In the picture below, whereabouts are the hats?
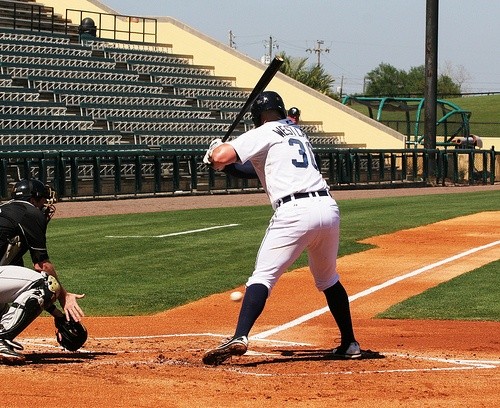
[288,107,300,117]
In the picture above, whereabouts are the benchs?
[0,0,377,180]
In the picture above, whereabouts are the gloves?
[203,138,225,171]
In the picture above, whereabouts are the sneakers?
[332,338,362,358]
[203,335,248,365]
[0,338,25,365]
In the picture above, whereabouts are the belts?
[278,191,332,207]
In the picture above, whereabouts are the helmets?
[11,177,52,199]
[251,91,287,128]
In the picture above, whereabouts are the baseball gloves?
[54,312,88,351]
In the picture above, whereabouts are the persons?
[288,107,300,124]
[0,178,85,364]
[199,91,362,367]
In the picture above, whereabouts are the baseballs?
[230,291,243,302]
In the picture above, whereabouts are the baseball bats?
[207,56,285,169]
